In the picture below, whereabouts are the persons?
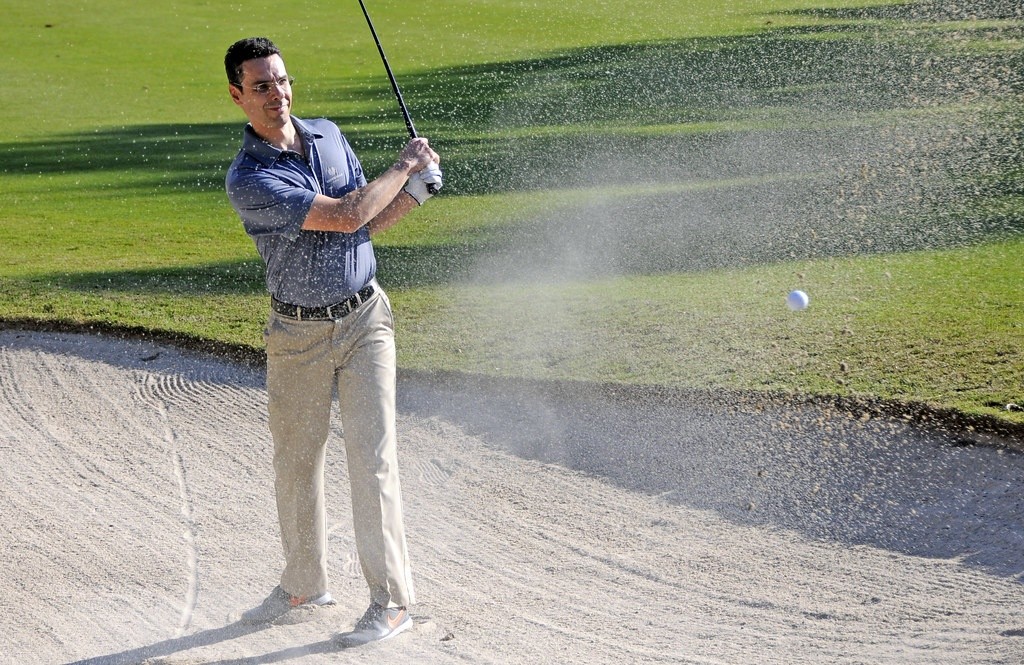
[225,38,443,648]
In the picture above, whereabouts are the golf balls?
[787,290,809,311]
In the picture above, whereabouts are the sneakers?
[340,600,411,647]
[242,581,332,626]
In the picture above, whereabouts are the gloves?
[404,159,443,206]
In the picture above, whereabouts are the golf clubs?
[358,0,442,196]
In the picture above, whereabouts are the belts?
[271,286,375,320]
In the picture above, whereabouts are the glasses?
[230,75,298,99]
